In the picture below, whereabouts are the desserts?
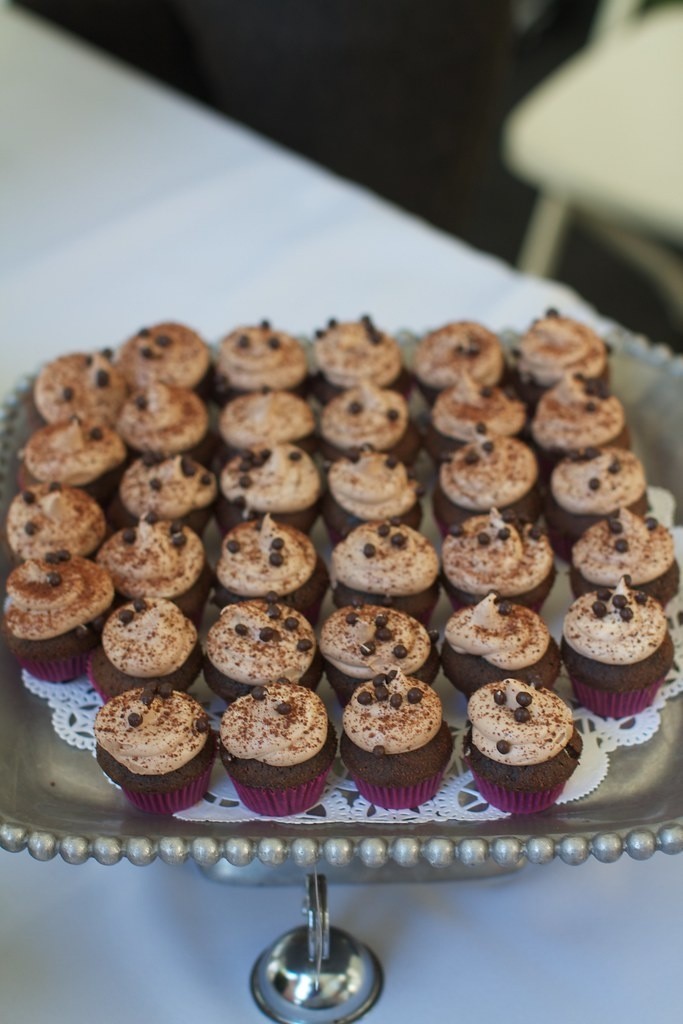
[0,307,680,813]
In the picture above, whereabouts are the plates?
[0,374,683,867]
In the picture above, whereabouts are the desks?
[0,0,683,1024]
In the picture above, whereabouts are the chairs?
[499,0,683,310]
